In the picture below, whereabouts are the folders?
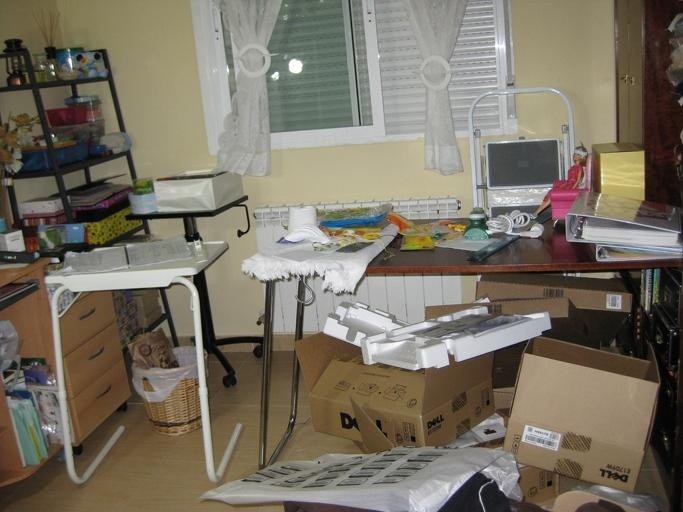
[565,190,682,247]
[595,244,683,263]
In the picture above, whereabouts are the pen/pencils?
[123,246,130,266]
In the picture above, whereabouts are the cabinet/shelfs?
[613,0,683,207]
[59,290,132,444]
[0,48,179,352]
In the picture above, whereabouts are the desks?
[43,241,243,486]
[124,193,248,389]
[248,203,682,471]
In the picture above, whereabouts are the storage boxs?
[503,336,661,494]
[154,168,243,212]
[76,200,143,244]
[472,406,560,503]
[424,298,598,386]
[592,142,645,200]
[295,332,496,447]
[476,272,633,313]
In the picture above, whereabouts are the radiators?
[252,196,462,336]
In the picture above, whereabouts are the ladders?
[467,85,577,284]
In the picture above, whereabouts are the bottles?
[33,52,47,83]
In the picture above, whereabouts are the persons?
[529,145,588,220]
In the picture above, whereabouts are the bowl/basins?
[45,107,87,128]
